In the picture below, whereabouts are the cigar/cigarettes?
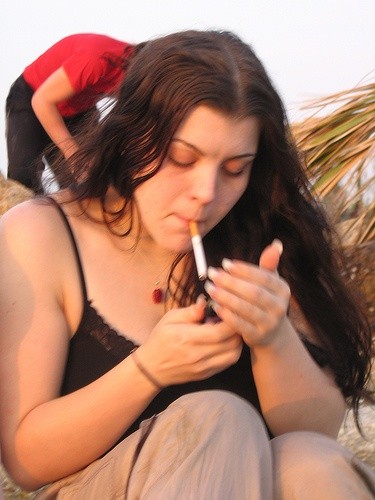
[189,221,208,280]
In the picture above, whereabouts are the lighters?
[205,298,220,323]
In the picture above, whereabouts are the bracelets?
[129,347,165,391]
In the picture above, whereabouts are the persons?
[5,33,136,195]
[0,29,375,500]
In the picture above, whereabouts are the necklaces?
[113,201,176,304]
